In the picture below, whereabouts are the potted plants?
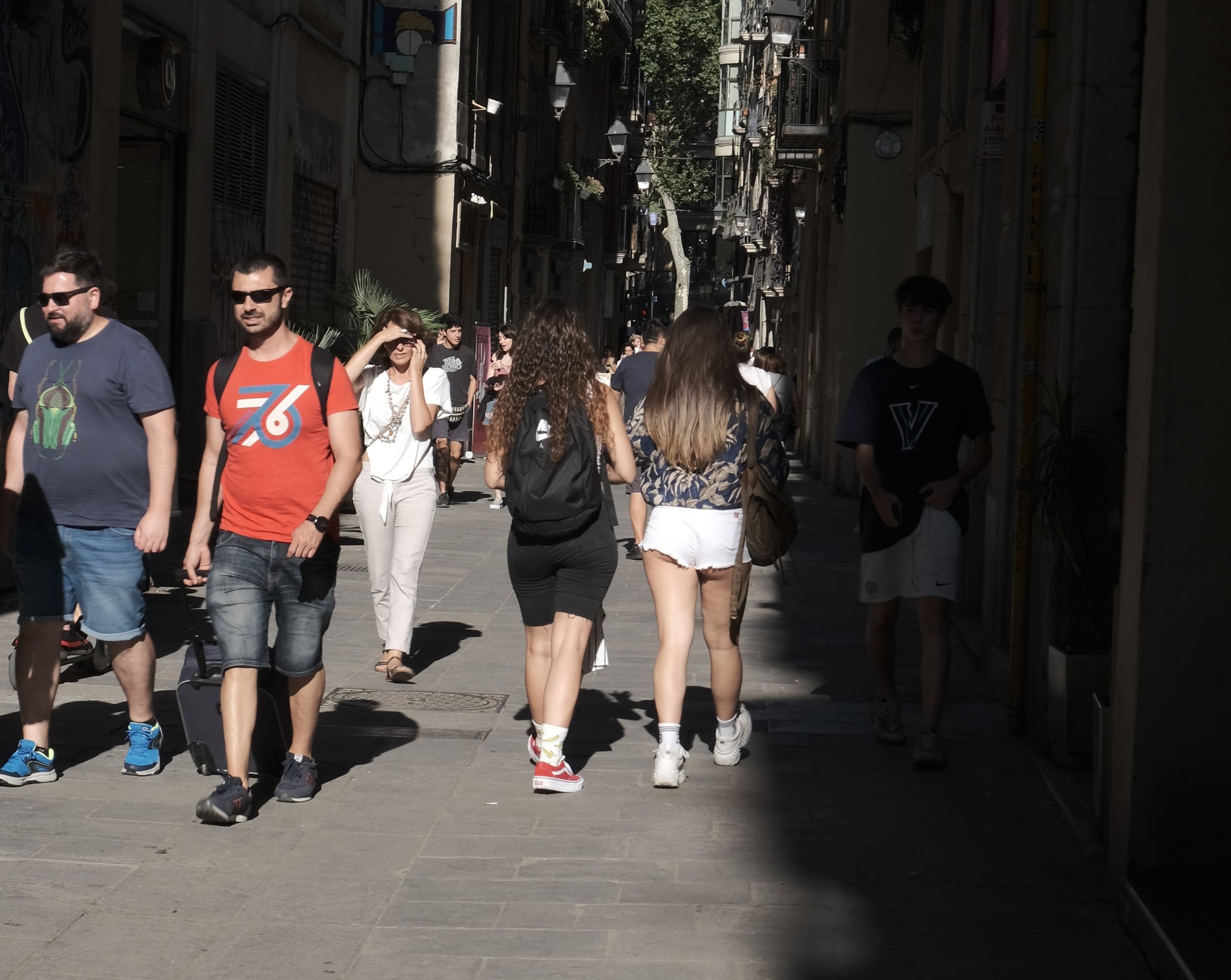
[647,201,664,226]
[553,163,580,190]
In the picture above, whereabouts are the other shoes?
[625,543,643,560]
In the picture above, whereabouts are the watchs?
[307,514,330,533]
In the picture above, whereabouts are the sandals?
[386,655,414,681]
[374,644,388,673]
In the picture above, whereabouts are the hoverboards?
[8,613,111,693]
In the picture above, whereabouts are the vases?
[580,188,591,201]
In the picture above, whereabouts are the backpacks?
[503,374,620,539]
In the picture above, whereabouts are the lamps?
[514,58,578,132]
[712,204,725,227]
[633,157,655,197]
[581,114,631,172]
[709,269,718,285]
[471,98,504,115]
[732,206,758,235]
[762,1,840,81]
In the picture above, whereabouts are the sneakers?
[489,497,503,509]
[196,768,253,823]
[446,486,457,504]
[652,741,690,788]
[436,489,450,508]
[0,739,58,787]
[527,732,583,793]
[121,719,164,777]
[274,751,318,803]
[60,632,94,655]
[911,722,947,770]
[714,701,752,767]
[869,695,907,745]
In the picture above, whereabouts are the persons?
[182,251,363,823]
[732,332,802,648]
[343,305,452,682]
[625,297,789,794]
[0,248,176,788]
[833,277,995,772]
[484,297,636,791]
[594,315,668,560]
[423,312,520,510]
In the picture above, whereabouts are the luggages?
[174,570,296,778]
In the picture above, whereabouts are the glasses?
[229,284,290,305]
[384,336,416,348]
[36,285,97,307]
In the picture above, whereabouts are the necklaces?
[380,370,415,442]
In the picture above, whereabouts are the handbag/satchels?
[445,403,468,423]
[729,384,800,620]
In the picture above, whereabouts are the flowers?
[583,176,604,200]
